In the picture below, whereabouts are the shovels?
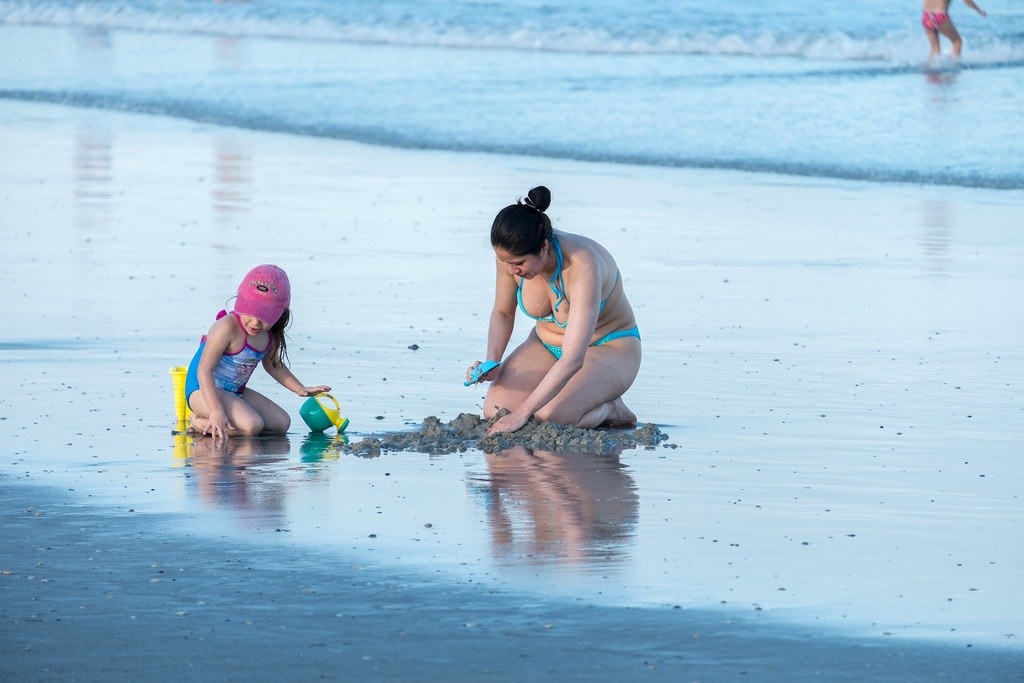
[464,360,501,387]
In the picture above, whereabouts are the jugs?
[300,392,351,433]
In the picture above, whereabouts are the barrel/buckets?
[170,364,192,421]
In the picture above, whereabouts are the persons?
[184,264,332,439]
[922,0,987,57]
[465,186,643,437]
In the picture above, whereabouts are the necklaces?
[531,251,557,324]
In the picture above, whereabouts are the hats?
[234,264,291,325]
